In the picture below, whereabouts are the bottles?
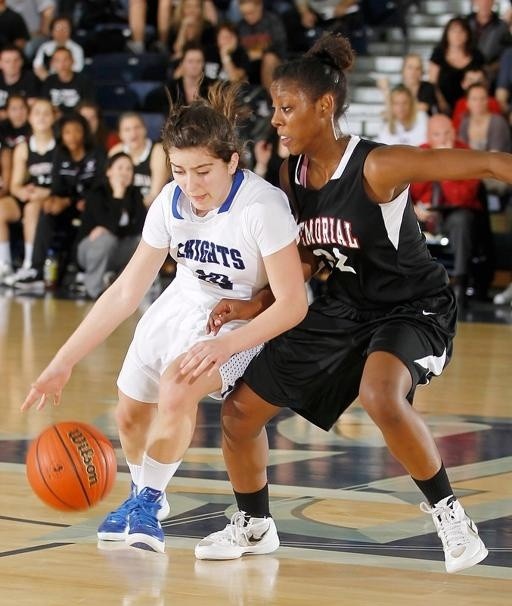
[43,249,58,287]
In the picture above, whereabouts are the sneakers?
[0,263,50,294]
[195,509,279,561]
[98,482,169,555]
[419,495,489,573]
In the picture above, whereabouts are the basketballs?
[26,423,117,511]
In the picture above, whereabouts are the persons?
[194,30,511,574]
[2,0,510,304]
[19,70,309,556]
